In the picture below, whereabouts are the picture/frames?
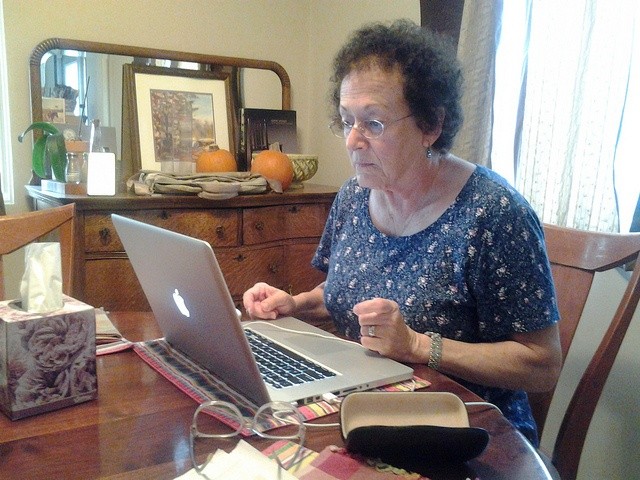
[121,62,237,176]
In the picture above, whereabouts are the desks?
[0,295,551,480]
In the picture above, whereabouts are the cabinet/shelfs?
[20,179,343,311]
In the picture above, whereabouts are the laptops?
[111,213,414,411]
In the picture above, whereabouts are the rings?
[369,325,375,338]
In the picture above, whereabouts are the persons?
[243,19,563,449]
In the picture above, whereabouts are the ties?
[328,112,415,140]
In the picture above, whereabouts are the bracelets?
[424,332,442,372]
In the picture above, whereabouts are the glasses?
[190,400,306,472]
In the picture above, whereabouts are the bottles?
[82,152,88,183]
[89,119,105,152]
[65,152,82,184]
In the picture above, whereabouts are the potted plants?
[17,120,91,182]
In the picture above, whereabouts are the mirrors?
[29,35,293,182]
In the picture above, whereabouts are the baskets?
[251,151,318,189]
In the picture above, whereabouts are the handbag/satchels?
[126,169,283,200]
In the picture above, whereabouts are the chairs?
[1,203,79,303]
[521,218,639,477]
